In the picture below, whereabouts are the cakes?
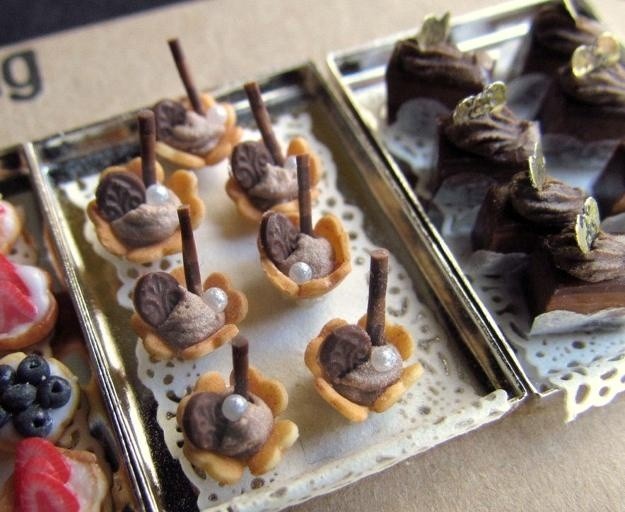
[128,204,249,361]
[0,349,80,453]
[594,143,625,219]
[424,80,543,195]
[0,251,58,353]
[385,10,497,128]
[256,153,352,301]
[225,81,321,221]
[86,110,205,263]
[518,195,625,319]
[0,436,112,511]
[152,37,243,168]
[468,143,600,256]
[177,335,300,485]
[521,1,625,75]
[537,29,624,123]
[0,196,40,267]
[304,249,425,424]
